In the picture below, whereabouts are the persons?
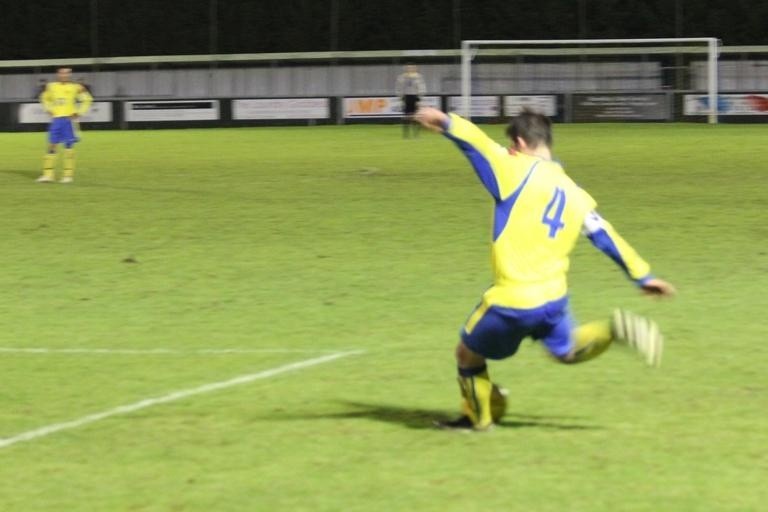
[395,66,427,139]
[414,105,678,429]
[35,65,94,183]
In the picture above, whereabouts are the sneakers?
[433,416,496,430]
